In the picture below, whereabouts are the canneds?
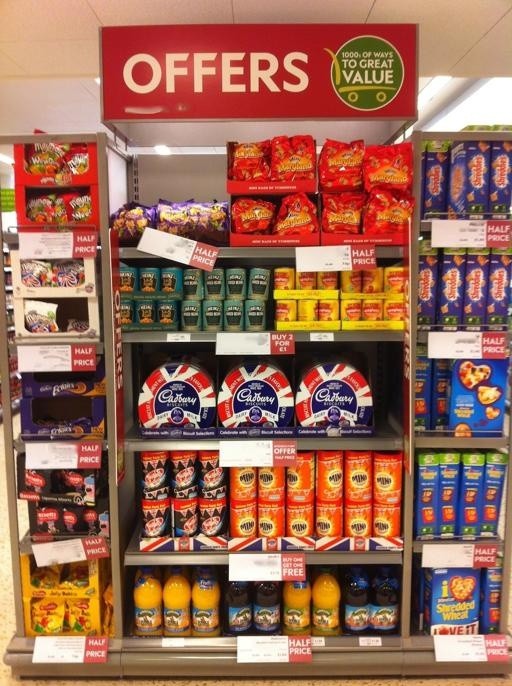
[119,265,406,332]
[141,451,401,539]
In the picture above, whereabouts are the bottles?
[132,568,399,636]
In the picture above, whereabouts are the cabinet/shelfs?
[0,128,511,681]
